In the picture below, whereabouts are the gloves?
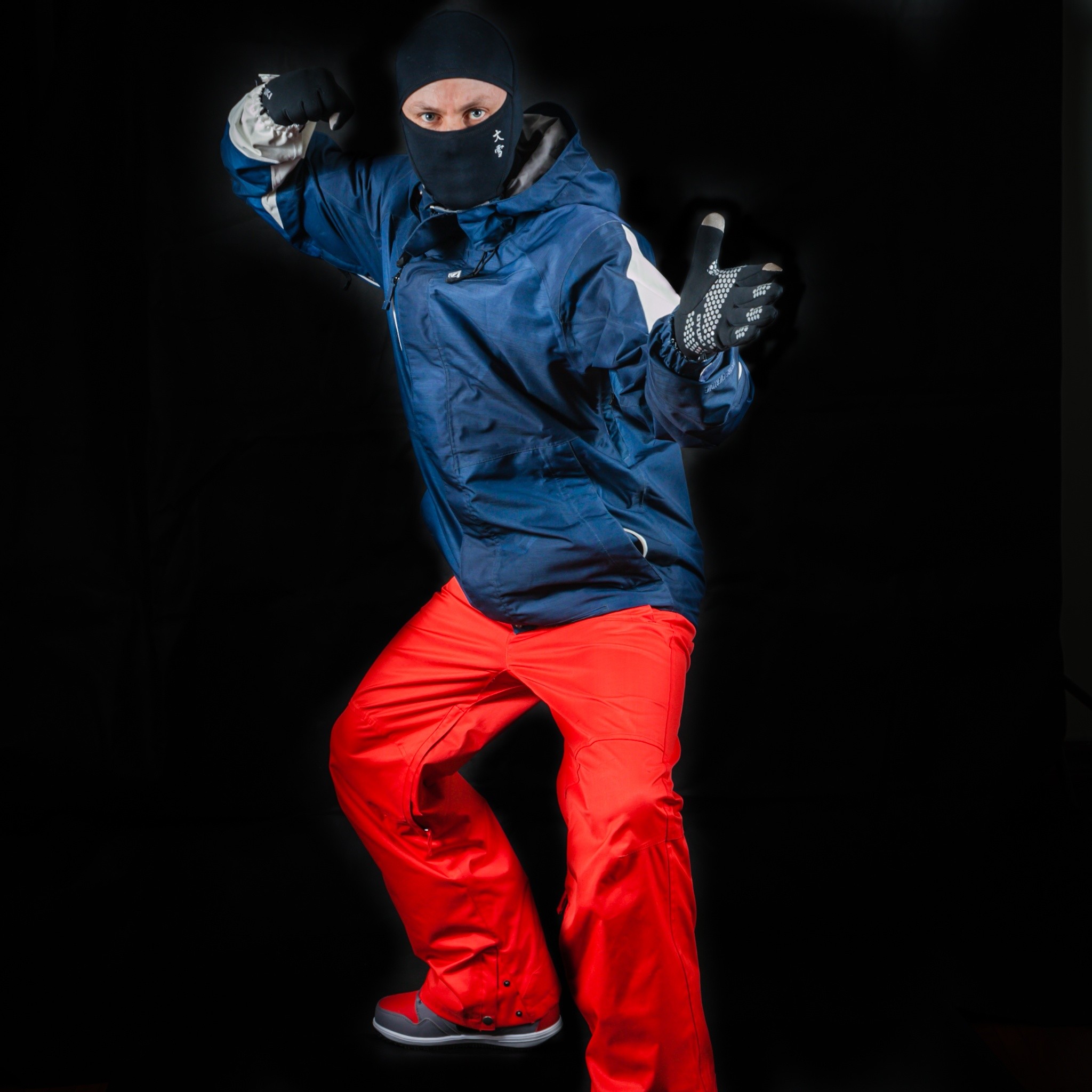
[673,211,785,360]
[262,67,351,131]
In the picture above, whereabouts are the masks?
[399,92,522,210]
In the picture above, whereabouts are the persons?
[219,9,783,1092]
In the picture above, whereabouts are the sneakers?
[372,989,564,1047]
